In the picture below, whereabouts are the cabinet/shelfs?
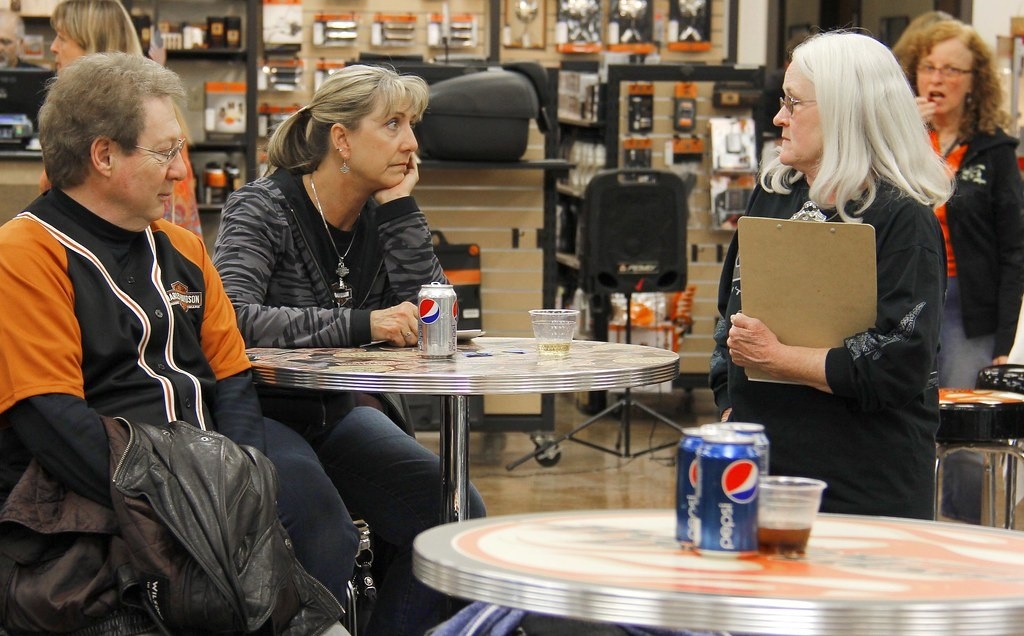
[121,0,258,211]
[555,64,762,414]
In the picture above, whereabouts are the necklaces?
[310,172,362,275]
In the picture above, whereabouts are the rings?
[403,331,412,337]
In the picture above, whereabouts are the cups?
[757,476,828,557]
[529,309,580,359]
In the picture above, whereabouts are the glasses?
[89,138,187,166]
[915,60,977,79]
[779,95,817,115]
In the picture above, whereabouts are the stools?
[932,388,1024,530]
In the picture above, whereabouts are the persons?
[0,11,42,73]
[213,65,488,636]
[1,53,353,636]
[711,31,954,521]
[38,0,203,238]
[892,9,1024,520]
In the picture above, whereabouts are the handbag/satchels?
[423,600,733,636]
[430,229,482,337]
[340,517,379,635]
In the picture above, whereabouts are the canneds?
[417,281,458,357]
[674,421,770,558]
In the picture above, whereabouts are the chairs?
[506,167,697,471]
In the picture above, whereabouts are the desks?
[411,508,1024,636]
[245,336,681,528]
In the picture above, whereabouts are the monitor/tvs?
[0,67,55,131]
